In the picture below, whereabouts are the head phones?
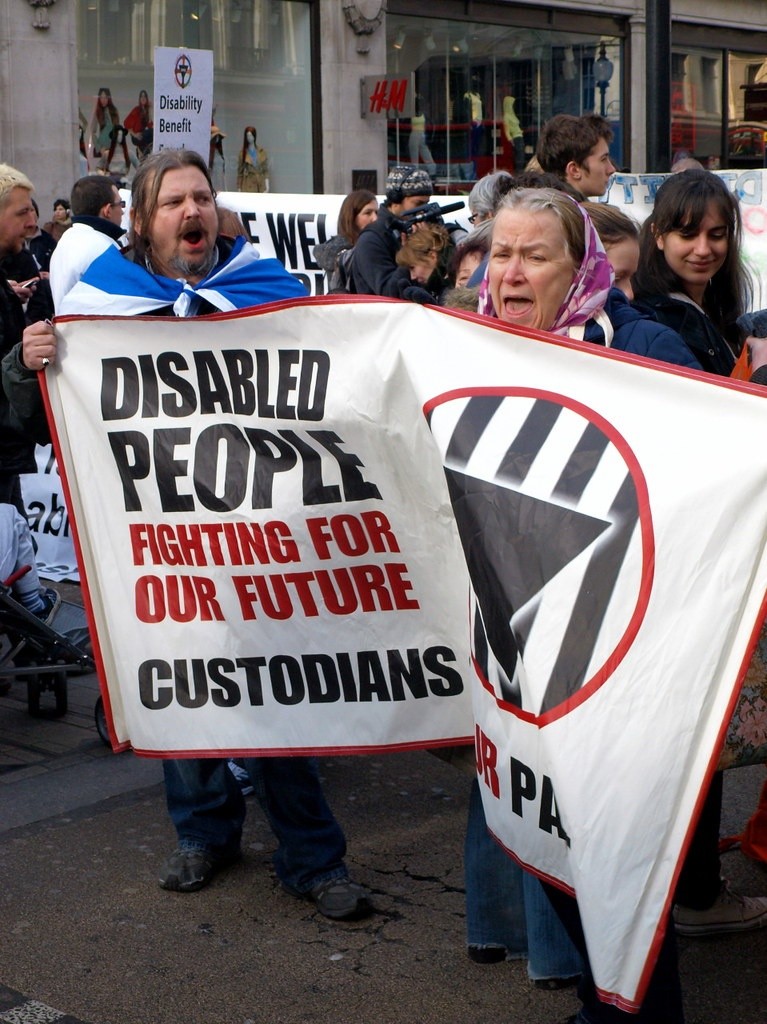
[387,166,416,205]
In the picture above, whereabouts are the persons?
[407,75,536,179]
[78,81,270,191]
[0,115,767,1023]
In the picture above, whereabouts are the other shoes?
[39,586,62,627]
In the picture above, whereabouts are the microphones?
[412,201,465,221]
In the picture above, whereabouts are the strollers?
[0,565,114,751]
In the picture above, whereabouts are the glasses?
[100,200,126,208]
[468,213,478,224]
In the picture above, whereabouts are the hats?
[386,166,432,197]
[31,198,39,217]
[210,126,226,139]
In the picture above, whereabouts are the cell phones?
[22,280,38,289]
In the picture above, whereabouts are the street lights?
[593,40,615,118]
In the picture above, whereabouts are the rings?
[42,358,49,367]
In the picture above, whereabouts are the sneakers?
[672,876,767,935]
[158,847,214,893]
[228,761,253,795]
[284,876,370,920]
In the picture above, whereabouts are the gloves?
[389,272,438,305]
